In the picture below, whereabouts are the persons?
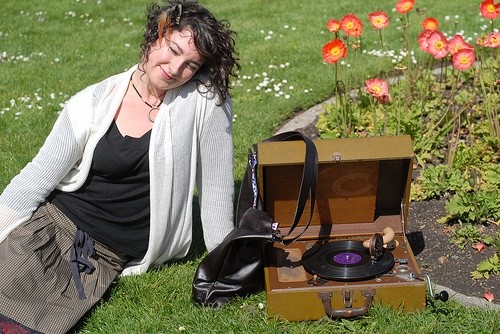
[0,1,242,334]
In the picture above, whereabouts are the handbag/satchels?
[191,130,318,308]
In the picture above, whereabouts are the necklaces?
[126,75,164,123]
